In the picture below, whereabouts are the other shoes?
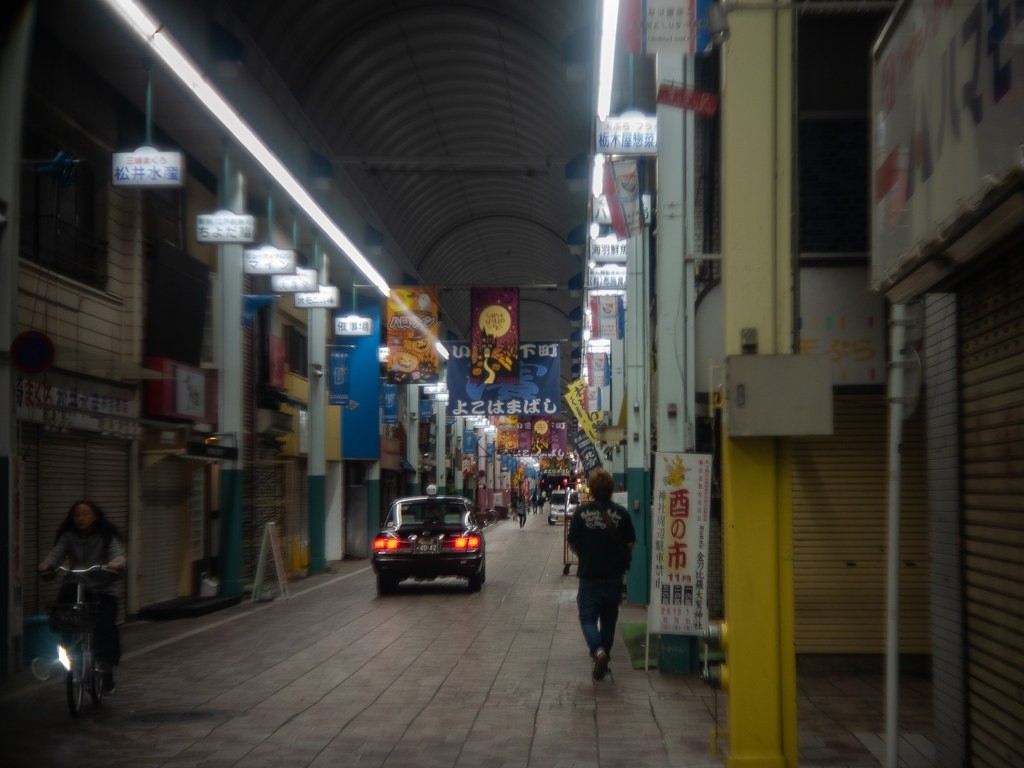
[593,653,612,681]
[103,672,115,696]
[520,527,525,531]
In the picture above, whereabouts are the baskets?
[48,601,101,632]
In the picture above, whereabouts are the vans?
[548,489,580,527]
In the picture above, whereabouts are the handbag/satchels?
[623,547,632,570]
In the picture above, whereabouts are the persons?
[566,468,636,681]
[38,499,125,695]
[528,491,547,515]
[517,495,527,531]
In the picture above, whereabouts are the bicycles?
[46,562,119,717]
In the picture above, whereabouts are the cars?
[370,494,489,596]
